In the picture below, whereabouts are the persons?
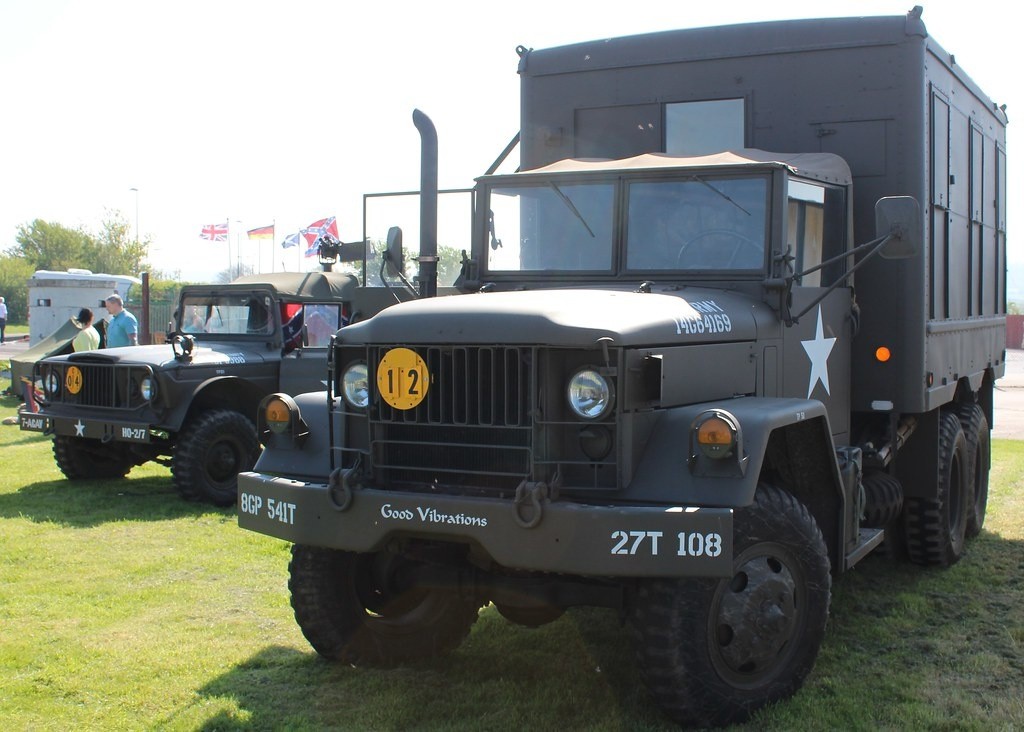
[105,295,137,348]
[72,308,100,353]
[0,297,7,343]
[174,305,205,332]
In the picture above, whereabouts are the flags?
[282,232,300,247]
[300,216,340,257]
[198,222,228,240]
[247,224,274,240]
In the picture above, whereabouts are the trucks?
[16,267,457,512]
[244,9,1008,710]
